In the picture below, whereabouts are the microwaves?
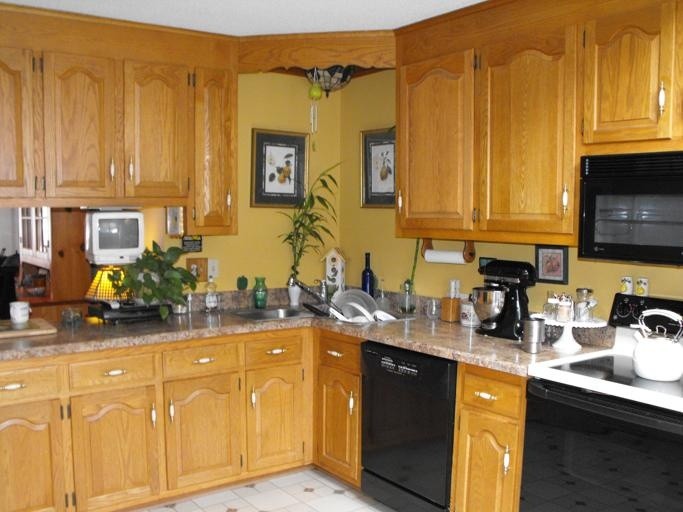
[576,155,681,263]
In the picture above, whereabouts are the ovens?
[518,379,681,511]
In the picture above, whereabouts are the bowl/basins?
[62,308,83,321]
[293,278,396,325]
[25,287,47,296]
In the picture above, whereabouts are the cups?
[8,301,30,324]
[513,316,545,354]
[460,299,479,327]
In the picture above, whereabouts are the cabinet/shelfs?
[313,330,363,488]
[447,363,526,512]
[1,355,67,512]
[360,339,457,512]
[13,206,93,301]
[241,331,308,476]
[167,30,238,237]
[575,2,683,156]
[66,335,244,512]
[1,4,45,203]
[44,8,188,208]
[394,0,580,246]
[518,379,682,512]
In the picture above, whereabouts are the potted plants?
[279,155,346,307]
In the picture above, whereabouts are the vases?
[253,277,267,308]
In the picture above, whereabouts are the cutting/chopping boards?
[0,317,59,339]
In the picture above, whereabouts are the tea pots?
[629,375,682,398]
[626,309,683,382]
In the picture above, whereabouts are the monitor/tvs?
[86,212,145,267]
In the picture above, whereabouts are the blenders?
[471,259,535,343]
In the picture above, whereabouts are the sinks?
[230,306,326,321]
[327,312,417,325]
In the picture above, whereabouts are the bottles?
[425,298,440,320]
[254,277,268,309]
[552,323,582,353]
[206,276,217,311]
[360,252,375,297]
[32,275,45,288]
[400,281,416,314]
[173,292,192,315]
[543,287,595,321]
[619,276,650,299]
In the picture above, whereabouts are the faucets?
[287,276,328,304]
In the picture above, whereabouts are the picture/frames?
[359,127,396,209]
[251,128,310,209]
[533,245,570,286]
